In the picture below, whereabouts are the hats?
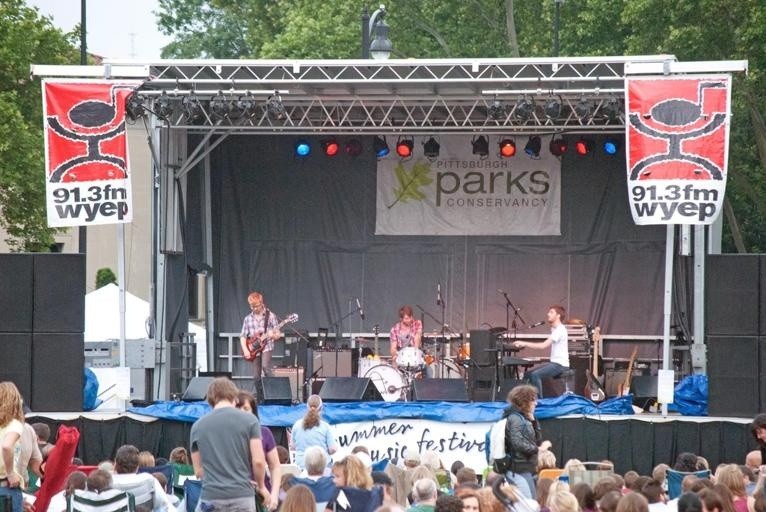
[404,453,420,467]
[434,470,451,494]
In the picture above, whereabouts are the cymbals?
[437,337,450,343]
[422,337,435,345]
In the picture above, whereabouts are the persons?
[31,423,55,485]
[326,445,524,512]
[234,390,281,511]
[507,385,543,505]
[524,448,765,512]
[1,382,25,512]
[751,413,765,465]
[239,291,280,405]
[512,305,570,398]
[290,395,338,472]
[264,446,325,512]
[362,347,379,360]
[389,306,422,401]
[188,377,265,512]
[17,403,44,512]
[49,445,193,511]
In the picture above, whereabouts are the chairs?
[665,469,712,500]
[538,469,566,482]
[558,474,568,483]
[136,465,175,495]
[183,478,204,512]
[567,462,614,484]
[65,488,136,512]
[275,463,301,488]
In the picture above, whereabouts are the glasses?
[250,302,261,308]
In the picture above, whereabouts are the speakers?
[706,336,759,418]
[258,376,292,406]
[0,253,33,332]
[33,252,86,333]
[319,375,384,402]
[411,377,470,402]
[704,253,760,335]
[32,333,84,413]
[631,374,658,402]
[180,376,216,402]
[0,333,31,411]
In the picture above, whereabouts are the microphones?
[436,282,442,304]
[356,300,366,321]
[528,321,546,328]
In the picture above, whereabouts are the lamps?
[470,134,622,161]
[292,134,441,164]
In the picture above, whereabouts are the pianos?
[469,326,534,401]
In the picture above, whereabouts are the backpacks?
[485,411,526,473]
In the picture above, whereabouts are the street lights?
[360,3,394,59]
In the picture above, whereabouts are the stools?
[541,369,575,399]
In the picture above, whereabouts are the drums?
[365,363,408,402]
[425,354,435,364]
[459,343,471,362]
[426,357,467,379]
[396,346,427,372]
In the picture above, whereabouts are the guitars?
[242,312,299,361]
[618,345,640,398]
[367,325,387,364]
[584,326,606,402]
